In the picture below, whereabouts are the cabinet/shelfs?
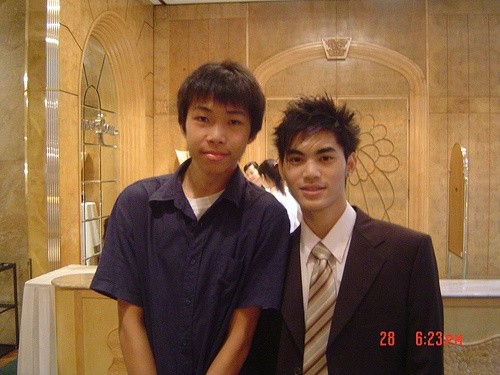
[0,263,19,359]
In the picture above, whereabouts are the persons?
[241,92,444,375]
[89,61,291,375]
[259,158,301,242]
[244,162,263,190]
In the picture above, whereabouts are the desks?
[17,264,98,375]
[81,202,100,265]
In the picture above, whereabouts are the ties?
[303,243,337,374]
